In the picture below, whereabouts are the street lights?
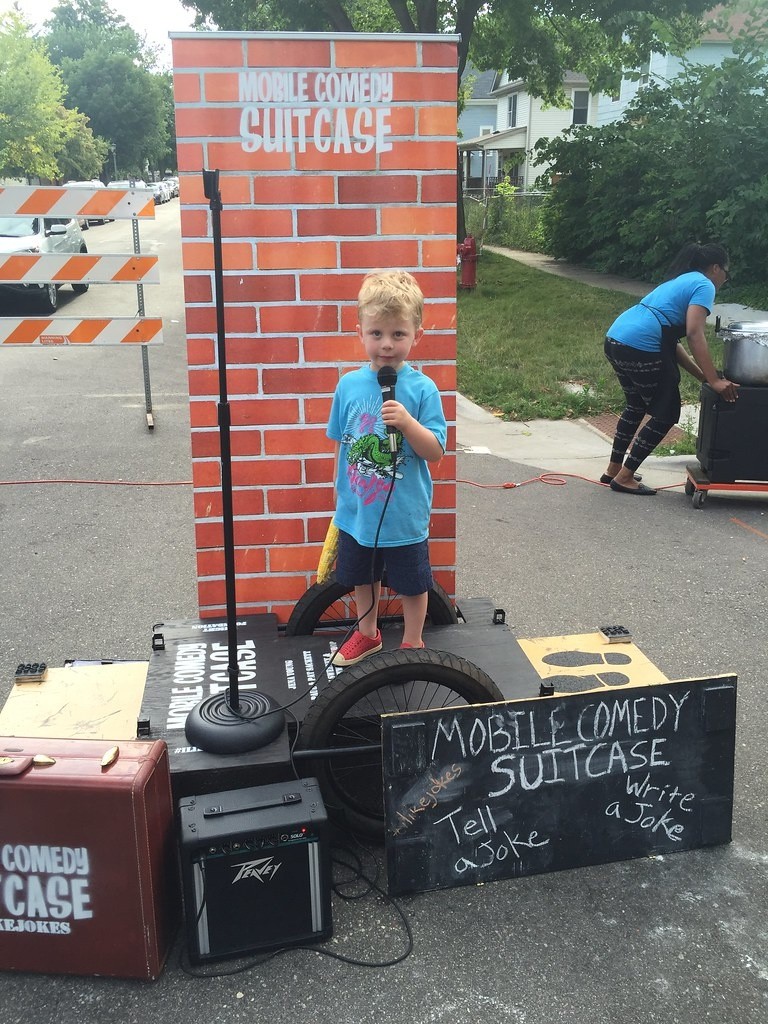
[110,142,118,181]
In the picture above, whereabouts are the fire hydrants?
[456,235,479,291]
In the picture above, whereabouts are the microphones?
[377,366,397,461]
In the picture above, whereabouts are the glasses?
[713,262,730,281]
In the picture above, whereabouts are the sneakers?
[399,640,426,649]
[330,630,384,666]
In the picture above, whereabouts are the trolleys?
[684,462,768,509]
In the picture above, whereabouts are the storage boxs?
[694,381,768,482]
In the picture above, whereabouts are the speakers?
[180,778,333,965]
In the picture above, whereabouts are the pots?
[716,313,768,387]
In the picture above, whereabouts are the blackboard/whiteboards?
[379,672,738,898]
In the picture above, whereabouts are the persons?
[325,268,449,682]
[601,242,742,496]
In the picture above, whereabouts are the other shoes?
[599,473,643,485]
[609,478,658,496]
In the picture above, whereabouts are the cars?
[61,176,179,231]
[0,216,92,314]
